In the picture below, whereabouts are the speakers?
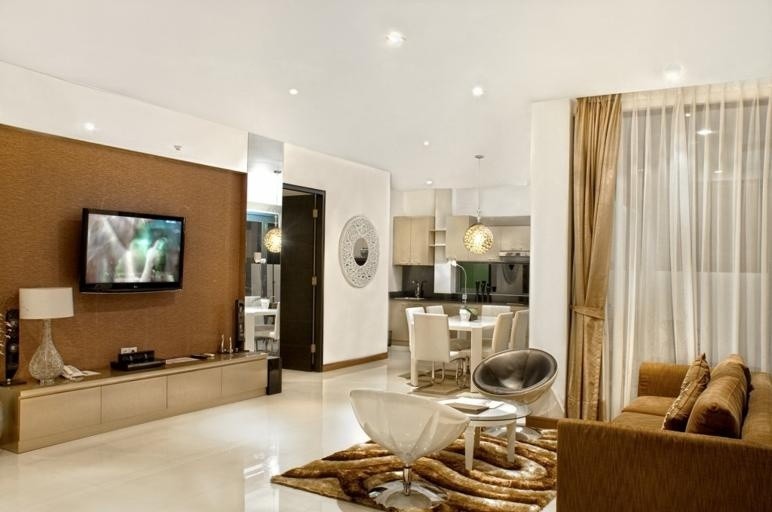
[0,309,27,387]
[234,298,249,352]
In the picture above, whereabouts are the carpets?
[270,429,559,512]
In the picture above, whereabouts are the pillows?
[725,352,752,386]
[661,352,711,431]
[684,362,749,440]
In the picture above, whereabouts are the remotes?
[204,352,215,357]
[191,354,207,359]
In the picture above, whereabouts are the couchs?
[556,361,771,512]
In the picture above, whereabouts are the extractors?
[499,251,530,264]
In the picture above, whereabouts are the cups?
[468,306,480,321]
[253,252,261,263]
[262,299,270,309]
[260,258,267,264]
[492,286,496,292]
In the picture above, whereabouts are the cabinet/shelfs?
[392,215,478,267]
[0,348,269,455]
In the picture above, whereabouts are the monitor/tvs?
[80,208,186,294]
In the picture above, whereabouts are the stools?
[471,347,558,443]
[349,388,471,511]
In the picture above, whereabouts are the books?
[446,401,488,415]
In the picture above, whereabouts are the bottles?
[456,295,470,321]
[227,336,233,353]
[476,279,491,293]
[217,329,225,354]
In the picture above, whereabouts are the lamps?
[463,155,494,257]
[263,228,280,255]
[19,286,75,387]
[448,258,468,304]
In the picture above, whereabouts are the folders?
[444,402,490,415]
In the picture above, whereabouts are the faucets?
[412,280,420,291]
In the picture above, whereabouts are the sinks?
[394,296,425,301]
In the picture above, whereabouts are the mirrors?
[339,216,380,289]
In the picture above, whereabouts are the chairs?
[405,304,529,388]
